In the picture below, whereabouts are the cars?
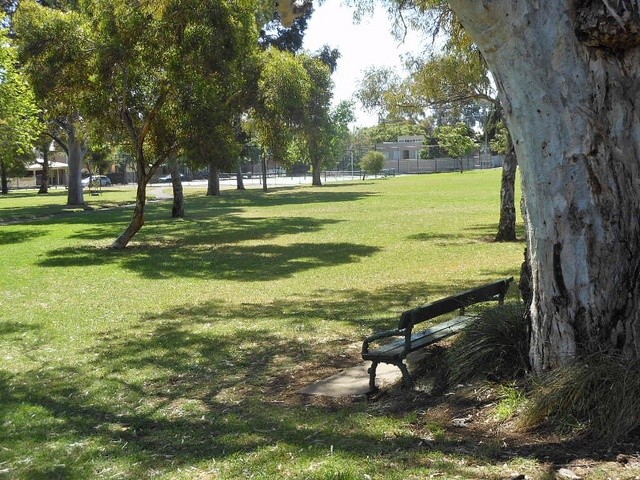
[158,172,185,182]
[81,175,111,187]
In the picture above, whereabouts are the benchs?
[361,275,514,388]
